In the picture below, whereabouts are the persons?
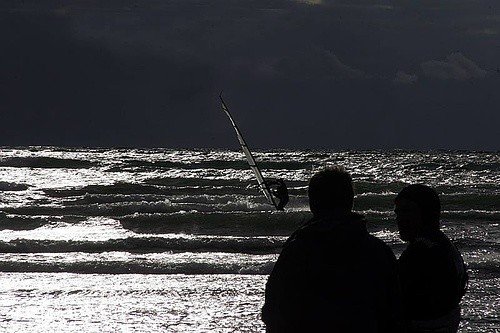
[272,176,289,212]
[394,185,467,333]
[261,167,403,333]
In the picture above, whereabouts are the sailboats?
[219,92,287,213]
